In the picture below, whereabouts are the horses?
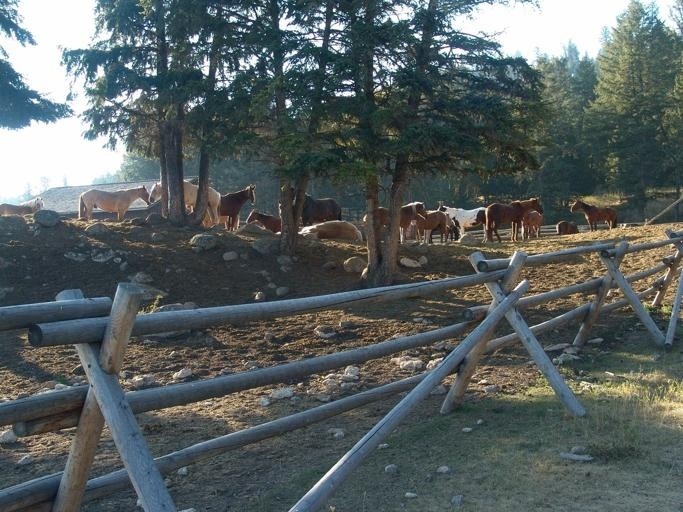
[78,183,149,221]
[363,196,544,247]
[0,195,43,218]
[569,200,618,232]
[146,177,256,231]
[299,220,362,245]
[556,220,579,234]
[290,188,342,224]
[245,208,282,233]
